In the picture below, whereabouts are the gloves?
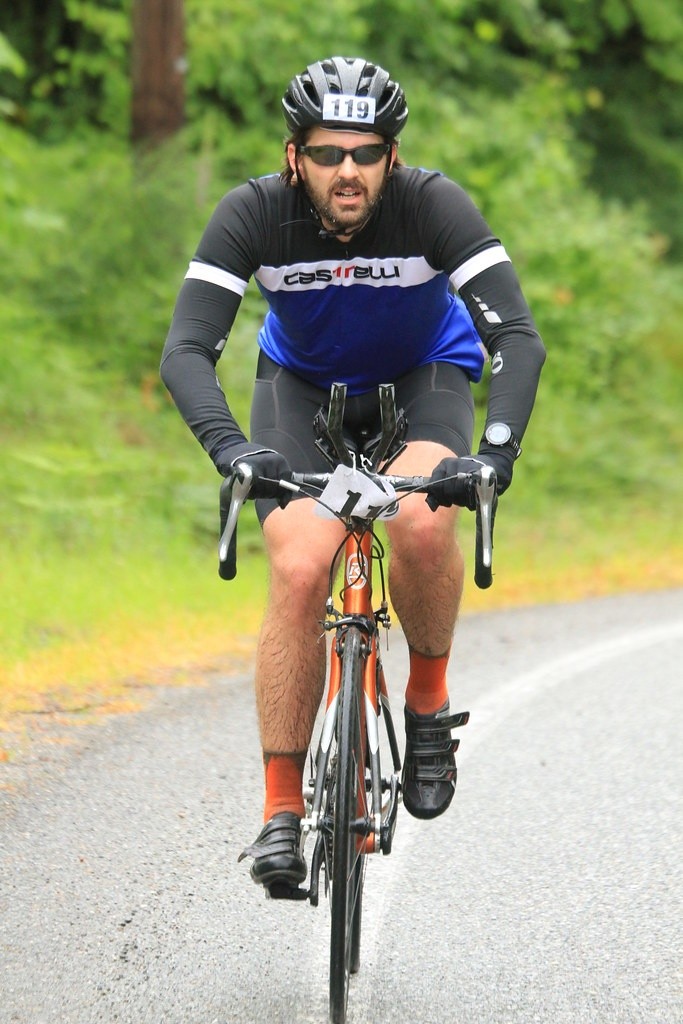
[428,454,497,513]
[216,442,292,510]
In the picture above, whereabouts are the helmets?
[280,56,409,141]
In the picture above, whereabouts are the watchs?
[479,422,522,458]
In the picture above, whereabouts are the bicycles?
[218,380,496,1024]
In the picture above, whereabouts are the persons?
[161,56,548,881]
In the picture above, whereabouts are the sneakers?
[400,697,470,821]
[237,811,308,884]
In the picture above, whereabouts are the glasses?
[295,142,393,167]
